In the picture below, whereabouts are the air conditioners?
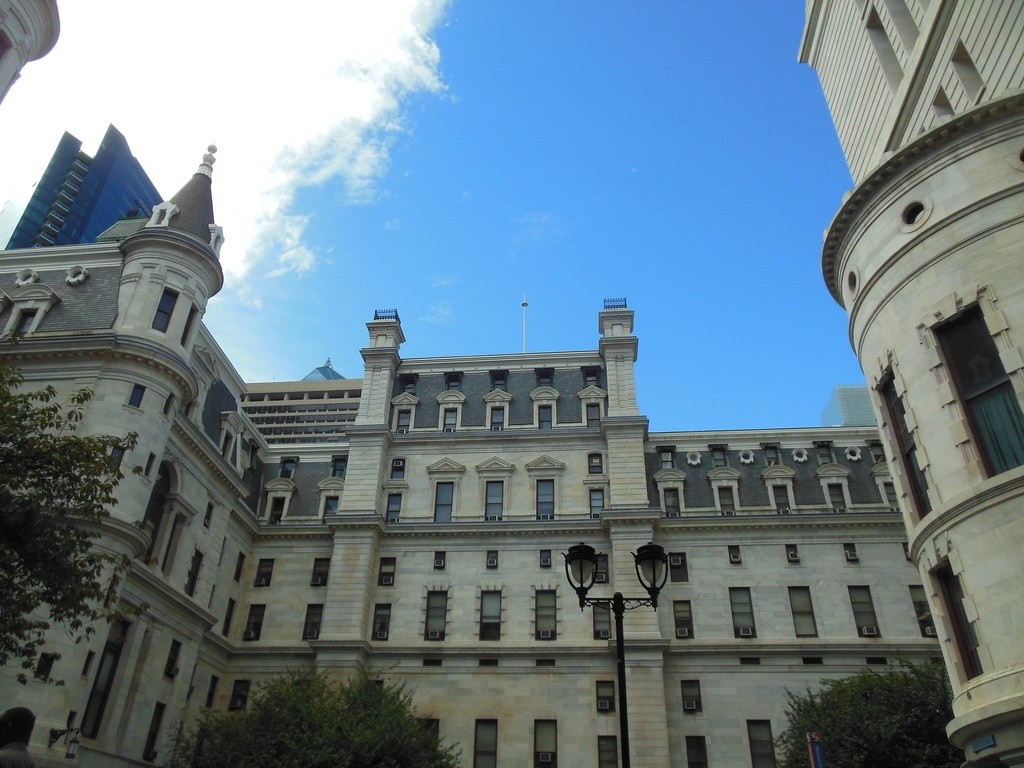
[847,552,857,559]
[444,427,454,432]
[671,558,681,565]
[862,627,876,635]
[780,509,790,514]
[311,577,321,584]
[595,574,605,581]
[230,699,241,708]
[168,666,179,675]
[730,554,740,561]
[835,508,847,513]
[541,558,550,565]
[668,512,679,517]
[270,520,279,525]
[540,514,550,520]
[492,426,501,431]
[540,630,550,638]
[788,552,798,560]
[383,577,392,584]
[397,429,406,434]
[429,630,439,638]
[539,753,551,763]
[255,578,265,585]
[598,700,609,709]
[388,518,397,522]
[487,559,496,566]
[394,461,402,466]
[600,630,610,638]
[678,628,688,637]
[306,630,317,638]
[684,700,695,710]
[591,513,600,518]
[739,628,752,635]
[377,631,387,639]
[892,507,899,511]
[926,626,937,635]
[146,751,158,759]
[244,630,256,638]
[489,515,498,521]
[434,560,443,567]
[723,511,734,516]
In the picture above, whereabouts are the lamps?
[48,726,80,759]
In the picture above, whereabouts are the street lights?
[564,538,672,768]
